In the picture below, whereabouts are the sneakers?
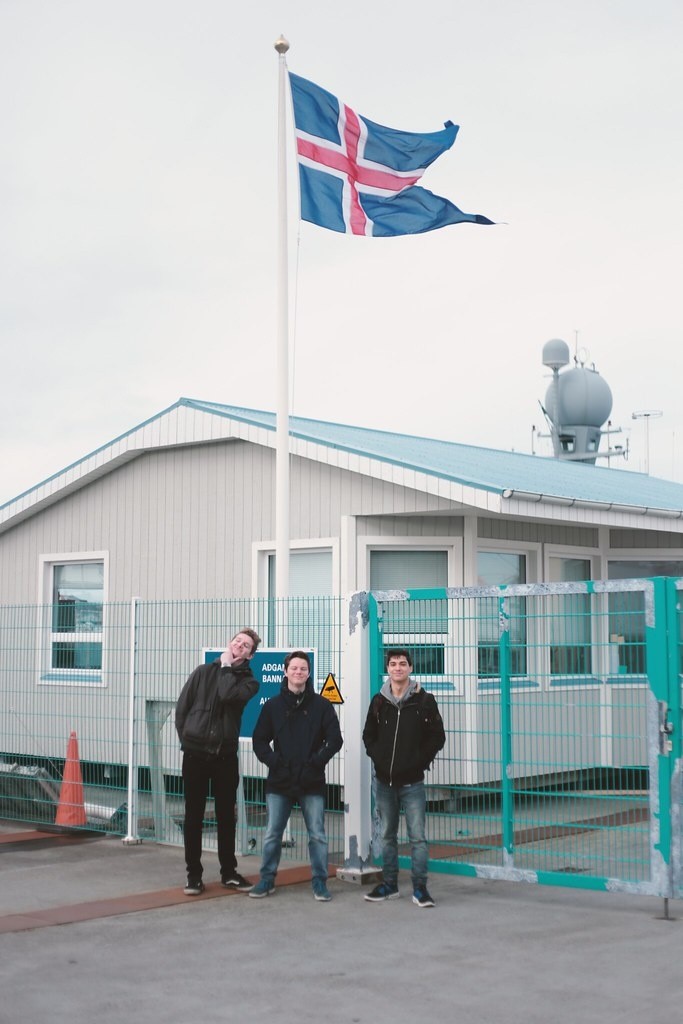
[364,881,400,901]
[413,888,435,907]
[184,880,205,894]
[313,884,332,900]
[249,879,276,898]
[220,874,255,892]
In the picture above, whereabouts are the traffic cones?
[36,730,106,836]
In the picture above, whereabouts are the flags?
[288,70,505,236]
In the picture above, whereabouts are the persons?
[362,648,446,907]
[176,628,262,895]
[249,651,344,901]
